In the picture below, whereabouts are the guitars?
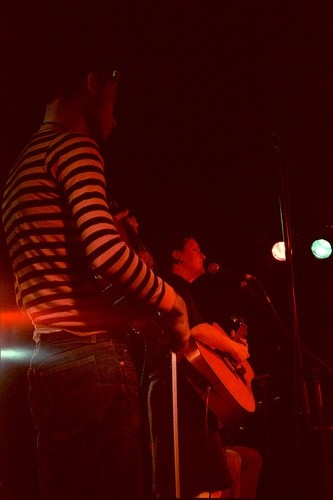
[175,315,257,424]
[95,194,174,355]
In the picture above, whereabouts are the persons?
[147,231,250,500]
[0,44,189,500]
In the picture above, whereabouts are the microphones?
[208,263,256,280]
